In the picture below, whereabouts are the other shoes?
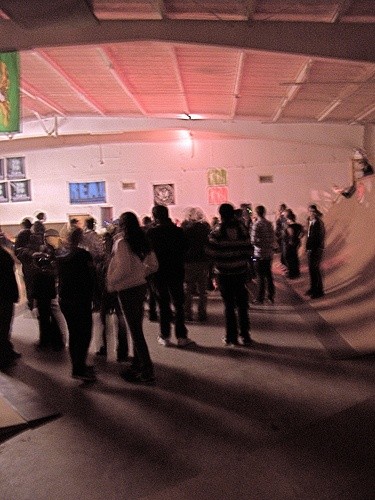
[72,372,98,383]
[176,336,190,346]
[221,335,234,348]
[240,335,251,345]
[157,335,170,347]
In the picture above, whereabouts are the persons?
[0,212,128,380]
[142,203,274,345]
[276,203,303,279]
[306,205,326,298]
[342,161,373,199]
[108,211,159,381]
